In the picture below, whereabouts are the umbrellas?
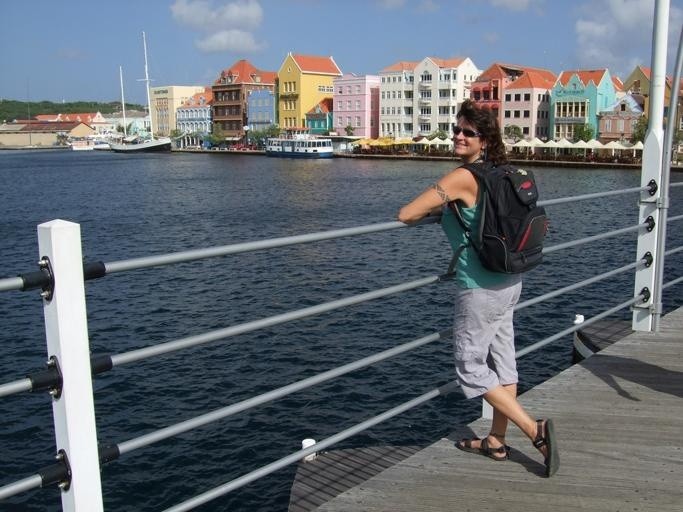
[351,137,644,162]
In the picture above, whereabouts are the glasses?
[453,126,481,137]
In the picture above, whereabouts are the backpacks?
[453,164,548,273]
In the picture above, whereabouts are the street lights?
[243,124,249,146]
[388,132,393,154]
[619,134,624,163]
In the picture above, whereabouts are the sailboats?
[104,29,170,153]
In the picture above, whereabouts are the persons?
[399,98,560,477]
[576,352,683,401]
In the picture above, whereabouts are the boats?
[264,137,333,159]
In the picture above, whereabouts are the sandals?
[455,438,510,461]
[533,419,560,477]
